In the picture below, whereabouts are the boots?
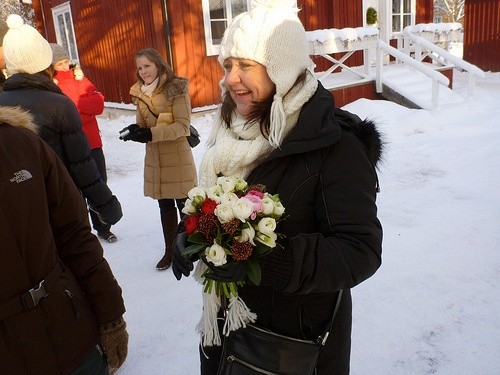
[156,207,177,270]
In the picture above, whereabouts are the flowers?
[180,170,291,298]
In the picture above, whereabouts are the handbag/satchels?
[188,125,200,148]
[217,310,326,375]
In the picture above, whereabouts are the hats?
[217,6,308,148]
[2,14,53,74]
[49,43,68,66]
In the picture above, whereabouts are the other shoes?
[97,230,117,242]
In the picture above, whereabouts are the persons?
[0,14,129,375]
[119,48,198,270]
[172,1,383,375]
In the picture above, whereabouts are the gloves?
[172,232,200,280]
[119,124,152,143]
[201,254,245,282]
[99,317,128,369]
[78,92,104,115]
